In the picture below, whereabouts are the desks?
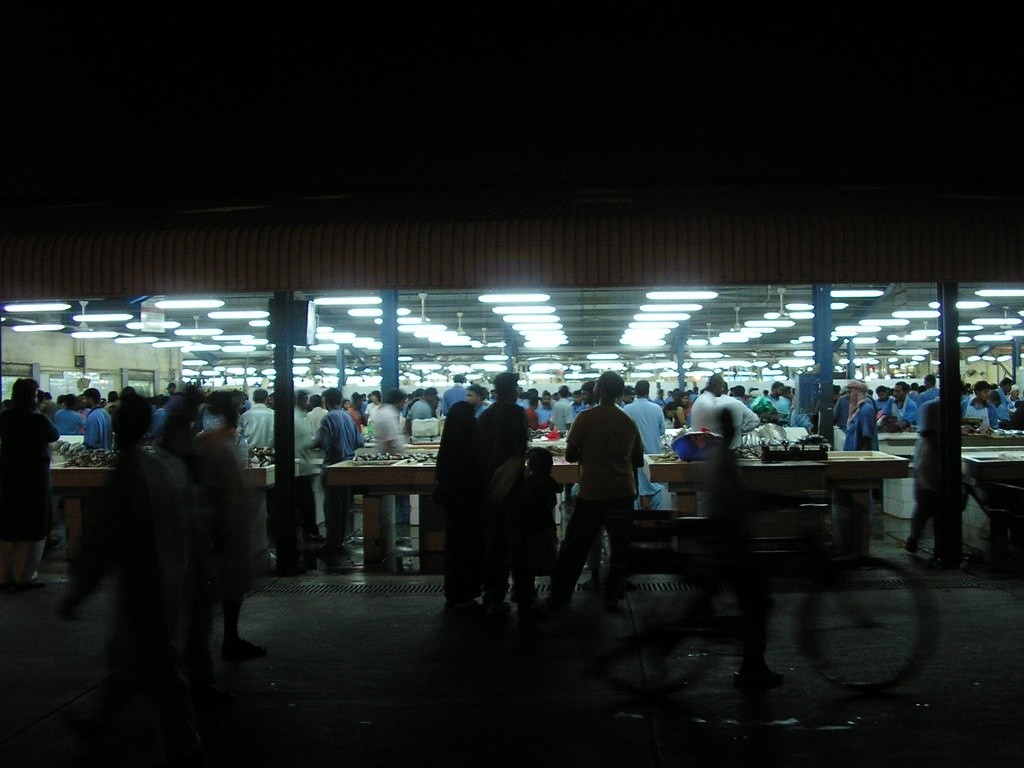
[48,431,1024,570]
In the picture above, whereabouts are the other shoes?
[906,537,919,552]
[194,682,240,708]
[482,600,510,612]
[520,602,546,617]
[16,581,40,589]
[220,637,258,658]
[605,600,619,611]
[1,580,14,589]
[540,597,569,610]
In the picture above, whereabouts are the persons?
[0,371,1024,732]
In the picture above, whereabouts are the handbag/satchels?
[490,456,521,499]
